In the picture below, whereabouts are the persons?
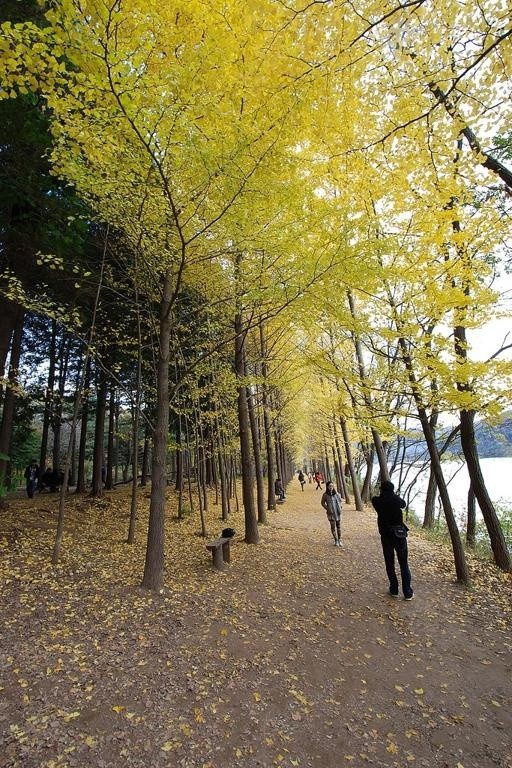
[274,478,285,500]
[307,470,322,491]
[298,470,305,492]
[371,480,414,601]
[24,458,68,500]
[320,481,345,547]
[101,462,108,485]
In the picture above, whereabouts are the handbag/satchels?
[393,525,406,538]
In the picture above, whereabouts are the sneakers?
[390,588,416,601]
[335,539,343,547]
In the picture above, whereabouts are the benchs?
[276,492,281,500]
[206,536,233,570]
